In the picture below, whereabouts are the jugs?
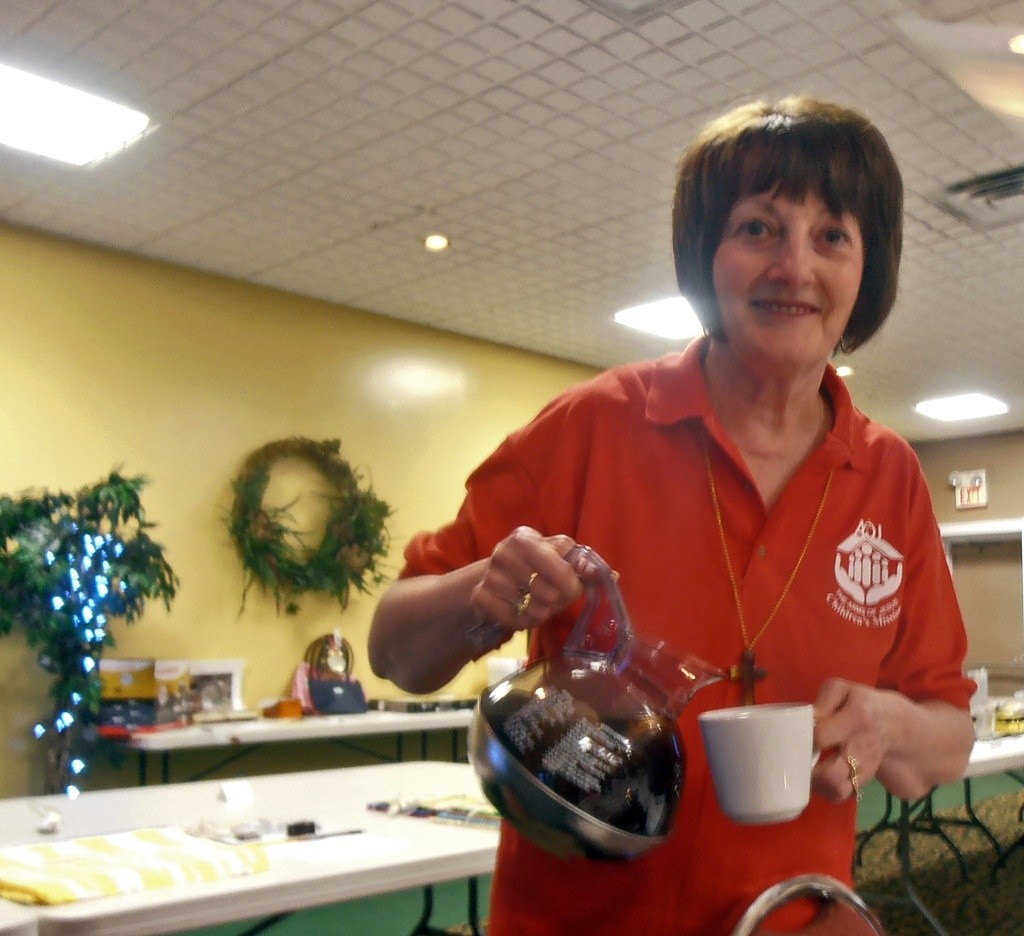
[468,544,729,861]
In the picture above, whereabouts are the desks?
[0,759,502,936]
[114,707,475,787]
[856,695,1024,884]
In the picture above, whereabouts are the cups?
[968,667,996,742]
[698,702,822,826]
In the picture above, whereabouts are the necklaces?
[699,422,833,707]
[739,393,824,459]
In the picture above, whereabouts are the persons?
[367,95,978,936]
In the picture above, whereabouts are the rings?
[527,571,539,588]
[512,587,534,617]
[847,754,864,802]
